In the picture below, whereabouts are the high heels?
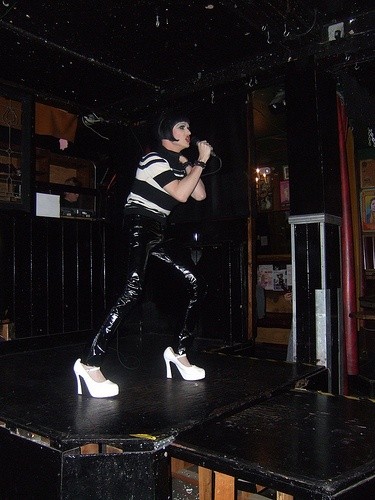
[74,358,118,398]
[164,347,205,381]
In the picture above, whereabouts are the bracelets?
[193,160,207,168]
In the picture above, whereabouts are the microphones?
[190,136,218,159]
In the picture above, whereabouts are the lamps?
[269,86,291,114]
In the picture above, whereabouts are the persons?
[73,102,215,398]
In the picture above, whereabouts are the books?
[257,264,292,291]
